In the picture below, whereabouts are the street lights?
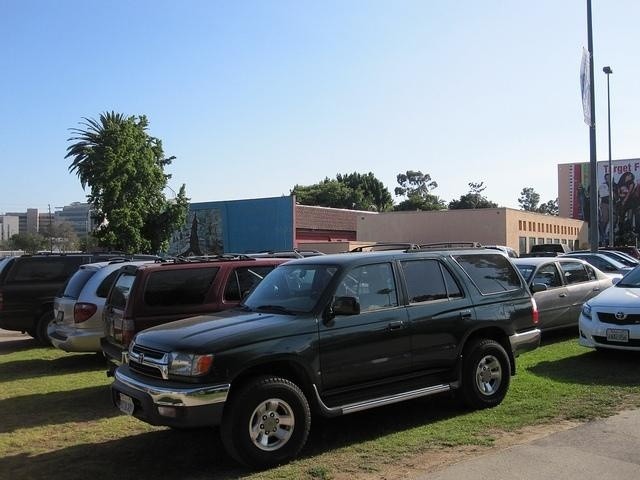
[602,64,616,248]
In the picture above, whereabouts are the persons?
[617,171,635,235]
[599,173,619,240]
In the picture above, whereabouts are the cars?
[493,246,640,355]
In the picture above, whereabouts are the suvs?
[47,259,206,359]
[1,252,168,344]
[107,242,541,469]
[100,252,357,380]
